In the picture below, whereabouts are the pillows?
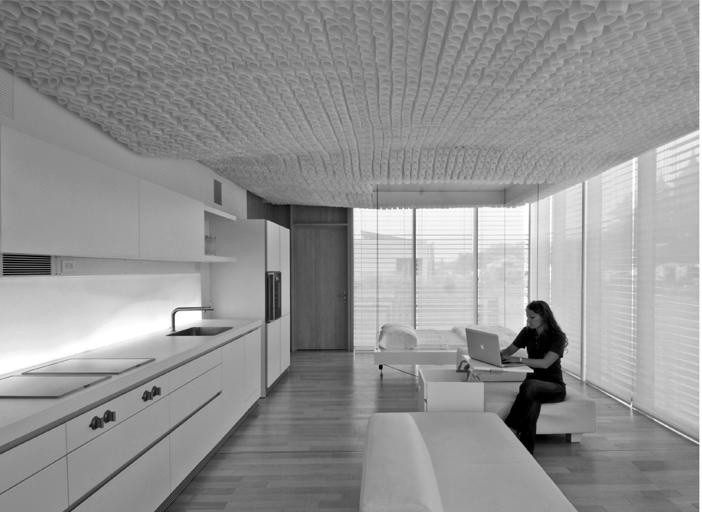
[378,324,418,350]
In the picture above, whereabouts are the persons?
[496,300,568,454]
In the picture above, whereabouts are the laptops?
[466,327,528,368]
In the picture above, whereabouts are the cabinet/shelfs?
[415,370,484,411]
[169,347,222,504]
[0,122,238,264]
[222,326,263,445]
[67,373,171,512]
[0,422,69,512]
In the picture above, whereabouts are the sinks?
[167,326,233,337]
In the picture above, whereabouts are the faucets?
[171,306,214,330]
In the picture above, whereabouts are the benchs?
[484,380,597,444]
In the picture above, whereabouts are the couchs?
[358,410,579,512]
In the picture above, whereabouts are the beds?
[373,324,527,378]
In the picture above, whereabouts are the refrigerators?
[206,217,293,398]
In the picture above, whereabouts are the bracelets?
[519,357,523,363]
